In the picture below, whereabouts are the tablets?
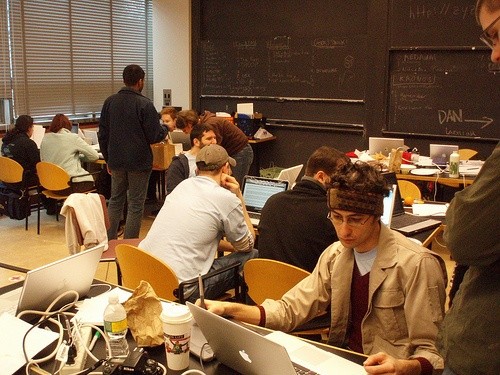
[0,245,106,322]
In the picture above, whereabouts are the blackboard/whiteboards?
[190,0,500,174]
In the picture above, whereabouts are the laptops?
[71,124,79,134]
[376,170,443,235]
[277,164,304,191]
[369,138,405,161]
[232,176,289,228]
[430,144,460,167]
[168,132,192,151]
[187,302,371,375]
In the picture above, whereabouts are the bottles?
[104,295,130,363]
[450,151,460,178]
[402,148,419,164]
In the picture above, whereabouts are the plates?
[368,156,483,176]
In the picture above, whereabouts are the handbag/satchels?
[0,185,31,220]
[152,133,176,170]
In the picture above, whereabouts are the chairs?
[35,161,103,236]
[59,192,144,287]
[397,180,422,201]
[0,155,59,231]
[435,149,478,201]
[114,244,248,306]
[241,257,331,347]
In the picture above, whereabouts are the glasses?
[479,17,500,48]
[327,212,373,226]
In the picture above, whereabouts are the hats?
[196,143,237,172]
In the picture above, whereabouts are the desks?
[81,150,190,219]
[0,276,382,375]
[350,154,486,185]
[217,198,449,259]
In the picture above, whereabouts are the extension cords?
[58,325,92,375]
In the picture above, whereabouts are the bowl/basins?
[429,144,459,165]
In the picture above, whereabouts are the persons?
[436,0,500,375]
[195,159,448,375]
[0,107,345,305]
[99,64,177,242]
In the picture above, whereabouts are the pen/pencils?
[198,274,206,309]
[89,330,101,351]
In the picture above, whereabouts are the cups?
[159,305,193,370]
